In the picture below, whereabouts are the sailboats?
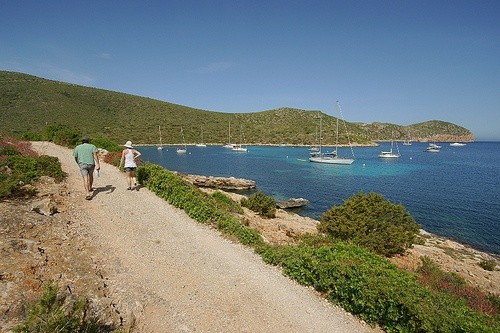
[190,126,208,147]
[177,126,188,154]
[305,100,357,165]
[224,119,249,153]
[157,125,165,151]
[378,130,403,159]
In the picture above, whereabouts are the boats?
[401,140,412,146]
[427,140,442,153]
[448,142,466,147]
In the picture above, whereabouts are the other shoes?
[89,188,93,191]
[85,196,92,200]
[127,183,139,191]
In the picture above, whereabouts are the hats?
[83,137,89,143]
[124,141,133,148]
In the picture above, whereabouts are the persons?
[73,136,100,200]
[119,141,141,190]
